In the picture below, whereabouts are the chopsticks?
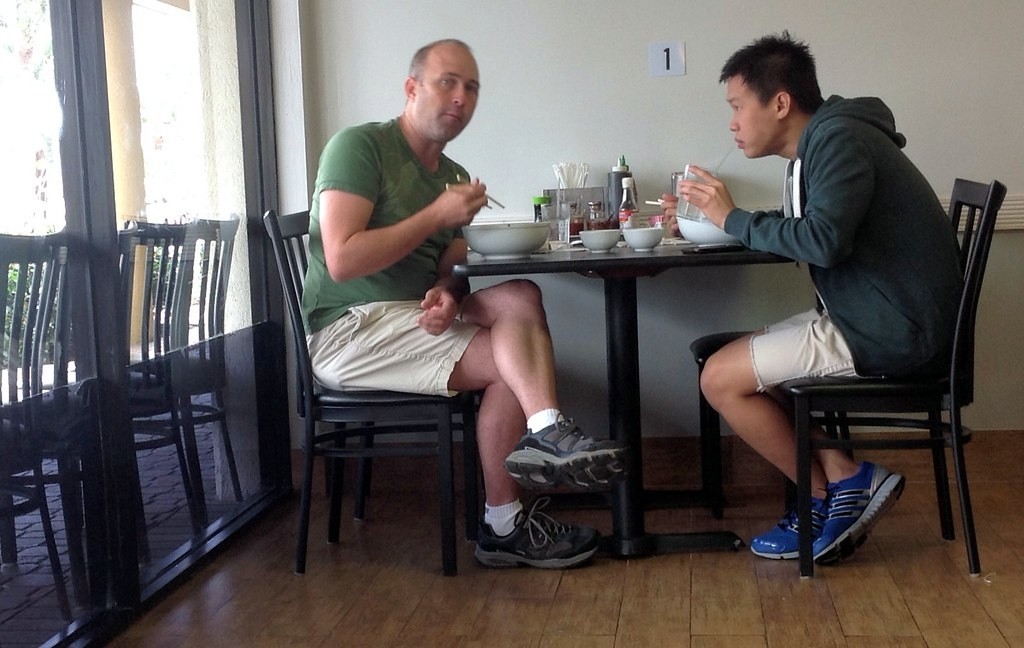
[445,174,505,209]
[554,162,588,189]
[645,198,664,206]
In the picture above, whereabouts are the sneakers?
[473,497,603,570]
[750,496,830,559]
[812,459,906,565]
[504,406,627,497]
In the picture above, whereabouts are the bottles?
[607,155,632,219]
[587,201,605,231]
[570,202,585,246]
[619,178,639,241]
[533,196,552,222]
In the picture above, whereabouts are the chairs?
[263,209,483,576]
[0,215,244,621]
[690,177,1008,578]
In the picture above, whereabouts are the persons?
[660,31,971,565]
[300,39,632,569]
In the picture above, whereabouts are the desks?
[452,243,797,560]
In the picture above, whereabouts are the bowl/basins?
[677,211,768,246]
[623,227,664,252]
[579,230,622,253]
[462,222,550,260]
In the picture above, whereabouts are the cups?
[676,165,718,222]
[541,204,570,251]
